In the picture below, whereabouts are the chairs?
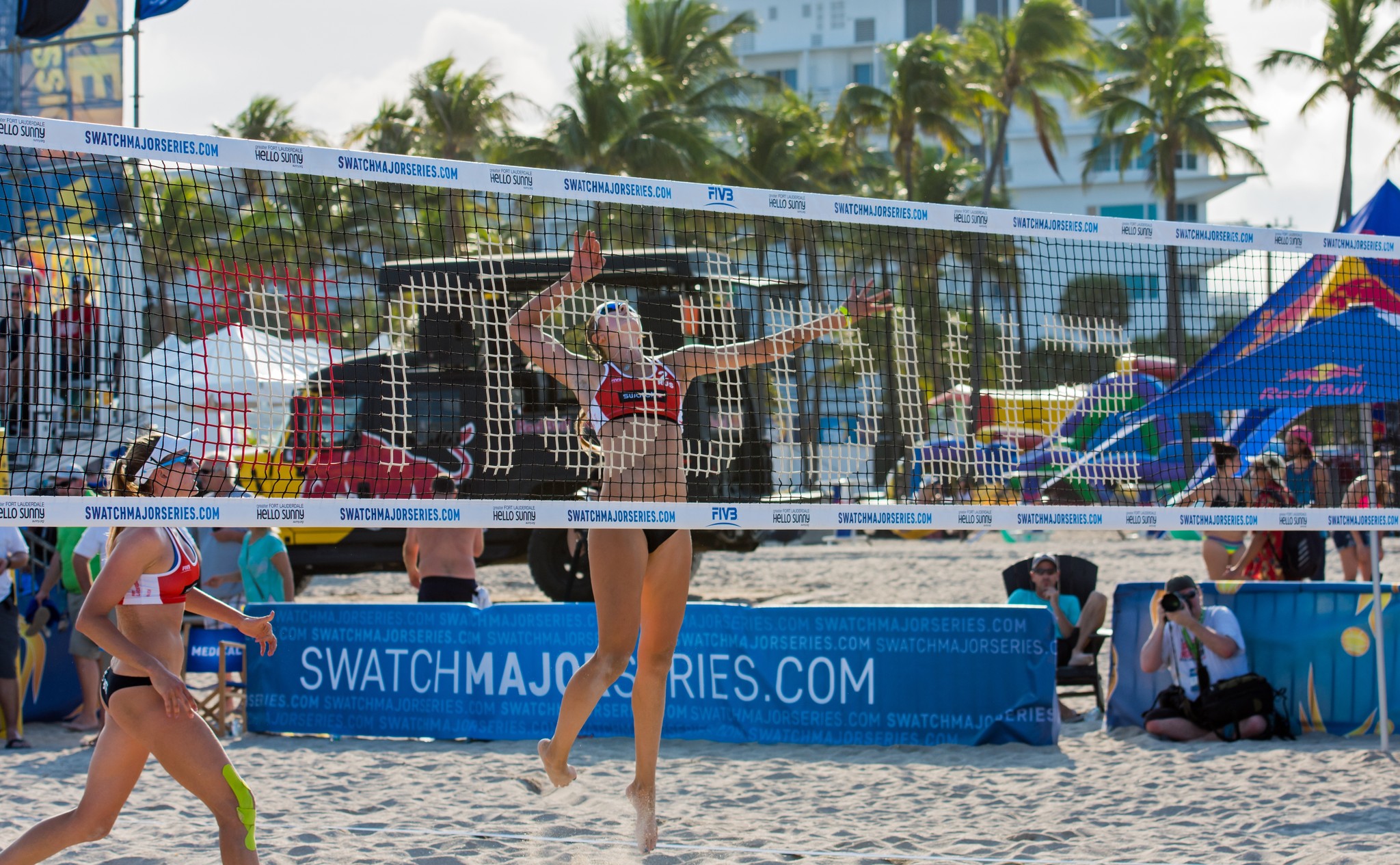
[180,624,247,736]
[1002,554,1112,712]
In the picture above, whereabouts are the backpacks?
[1257,488,1325,582]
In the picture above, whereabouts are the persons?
[0,428,483,865]
[506,229,893,853]
[0,273,99,403]
[906,426,1395,744]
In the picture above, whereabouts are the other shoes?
[26,606,50,636]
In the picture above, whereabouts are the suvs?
[288,242,775,607]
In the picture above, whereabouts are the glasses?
[1035,567,1056,575]
[57,477,77,487]
[593,301,638,333]
[73,289,81,295]
[10,291,26,298]
[1182,588,1196,599]
[199,469,215,475]
[142,452,194,473]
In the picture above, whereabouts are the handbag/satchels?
[1142,685,1192,728]
[1196,671,1294,738]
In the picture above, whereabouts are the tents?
[141,321,393,457]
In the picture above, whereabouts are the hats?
[1289,425,1313,451]
[1032,553,1057,570]
[1246,451,1287,488]
[72,275,89,288]
[1167,576,1198,592]
[134,428,199,486]
[46,463,88,480]
[432,475,455,493]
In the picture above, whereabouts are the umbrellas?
[1119,179,1400,751]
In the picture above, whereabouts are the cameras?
[1159,592,1187,612]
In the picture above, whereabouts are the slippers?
[1065,707,1102,722]
[5,739,28,748]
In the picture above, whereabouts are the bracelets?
[5,555,11,570]
[836,305,852,327]
